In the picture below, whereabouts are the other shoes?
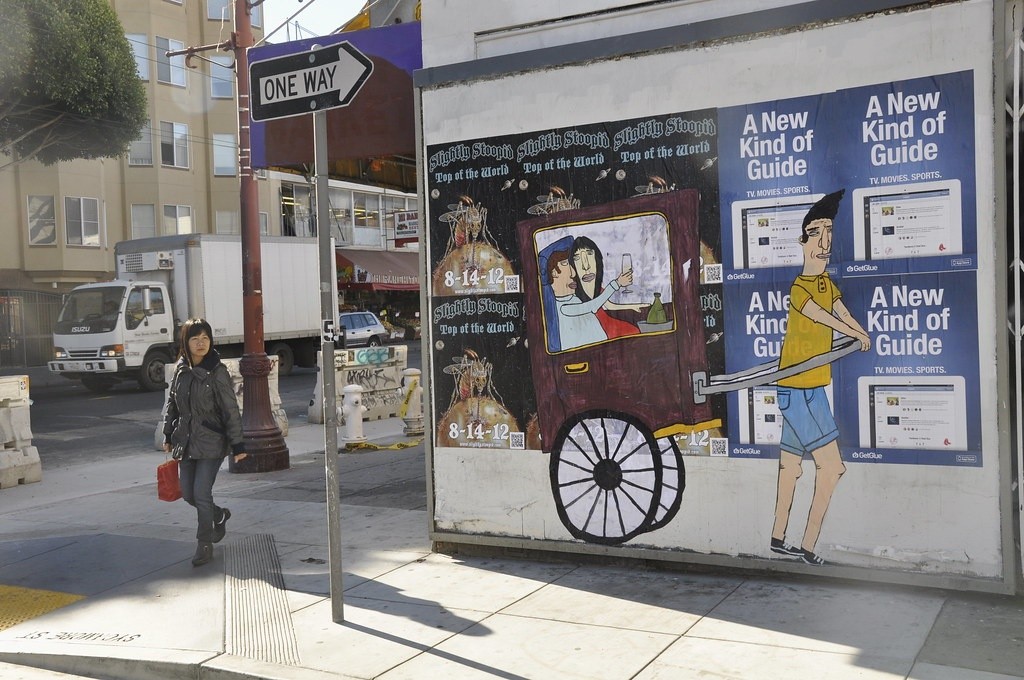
[212,508,231,543]
[192,542,213,565]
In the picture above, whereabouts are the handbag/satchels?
[157,450,184,501]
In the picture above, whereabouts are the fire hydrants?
[337,385,371,450]
[396,367,422,438]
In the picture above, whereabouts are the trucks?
[47,233,339,392]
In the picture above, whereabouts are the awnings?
[333,247,421,293]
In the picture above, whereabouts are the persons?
[104,300,117,312]
[163,317,247,566]
[127,295,144,324]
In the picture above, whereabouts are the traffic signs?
[248,40,374,124]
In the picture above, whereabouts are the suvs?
[338,310,387,347]
